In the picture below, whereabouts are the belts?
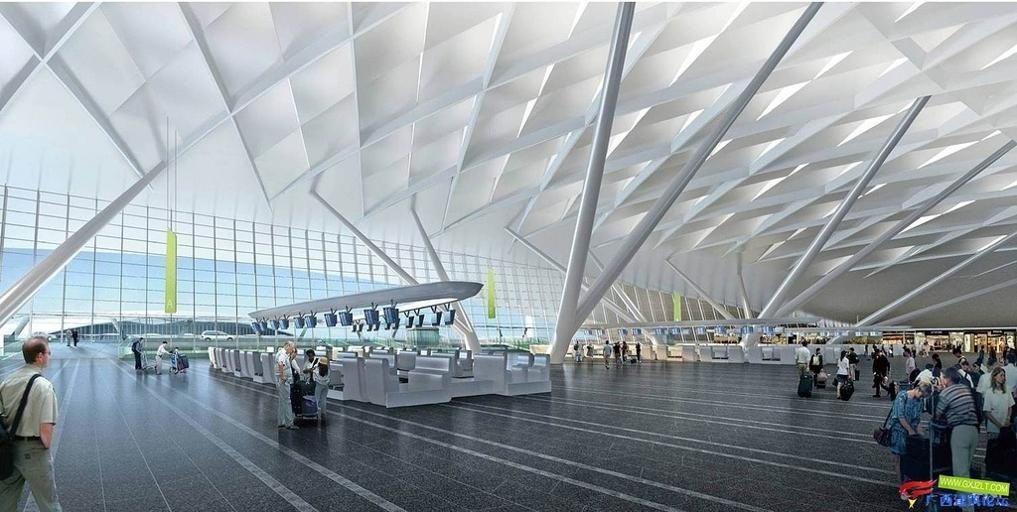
[12,434,41,443]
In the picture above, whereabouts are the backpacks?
[812,353,822,365]
[959,370,972,387]
[131,341,141,351]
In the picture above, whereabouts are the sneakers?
[871,385,891,398]
[277,423,300,431]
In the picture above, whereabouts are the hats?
[940,366,960,381]
[320,356,329,365]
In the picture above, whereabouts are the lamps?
[486,242,495,320]
[672,291,681,321]
[163,116,177,315]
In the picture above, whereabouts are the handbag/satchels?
[840,379,855,401]
[873,426,892,445]
[0,426,14,480]
[832,378,838,386]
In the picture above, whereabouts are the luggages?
[903,435,953,481]
[890,379,912,401]
[855,369,860,381]
[796,371,826,397]
[177,355,189,370]
[290,380,319,416]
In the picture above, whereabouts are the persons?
[132,336,145,370]
[571,339,642,369]
[72,327,79,346]
[273,340,330,431]
[0,336,64,511]
[168,346,189,374]
[66,327,73,346]
[155,341,174,375]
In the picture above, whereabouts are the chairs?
[586,341,906,366]
[206,343,552,409]
